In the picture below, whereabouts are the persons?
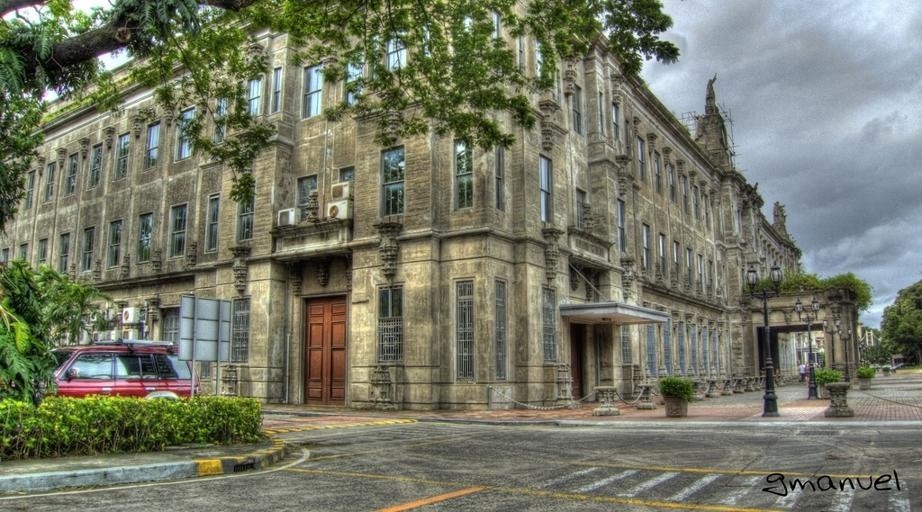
[799,363,820,383]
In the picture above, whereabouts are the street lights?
[746,261,784,417]
[836,328,853,383]
[823,315,840,369]
[795,295,822,402]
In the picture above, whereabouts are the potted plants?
[814,364,876,419]
[658,375,697,417]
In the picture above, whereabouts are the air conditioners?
[276,207,302,227]
[325,179,354,221]
[79,307,140,343]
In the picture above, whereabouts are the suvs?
[46,339,202,400]
